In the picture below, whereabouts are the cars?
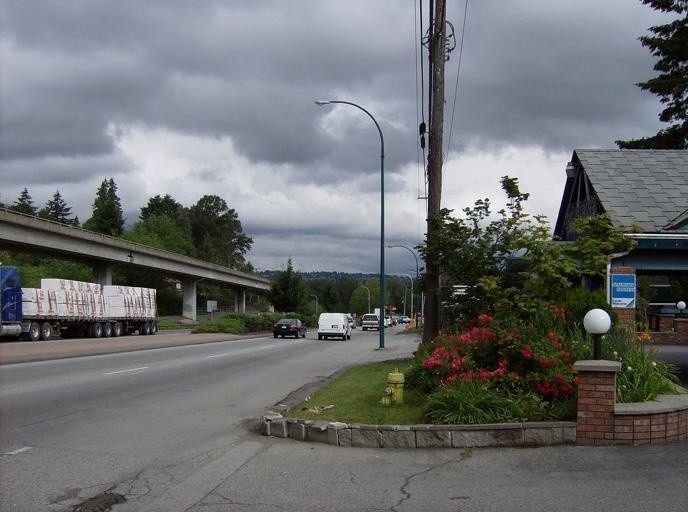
[318,312,356,340]
[272,319,306,338]
[362,314,411,331]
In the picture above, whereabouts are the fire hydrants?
[380,368,405,406]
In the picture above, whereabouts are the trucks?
[0,265,159,341]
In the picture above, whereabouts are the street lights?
[316,100,385,348]
[310,295,317,315]
[388,245,419,329]
[360,286,370,314]
